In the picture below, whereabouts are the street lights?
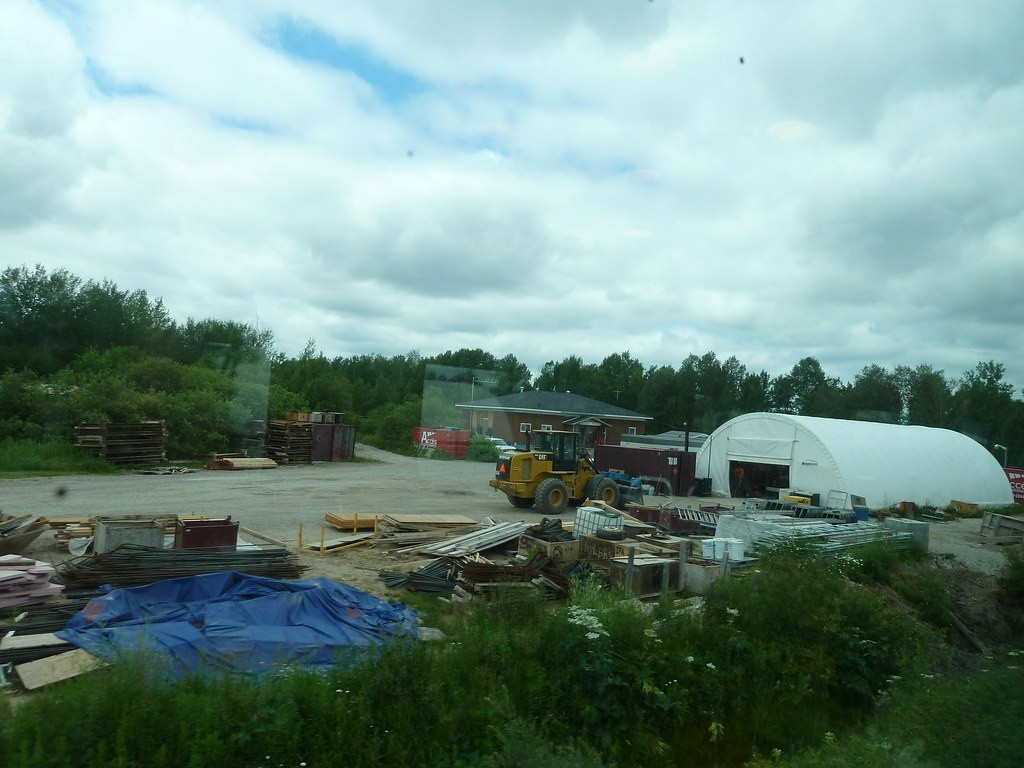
[995,443,1008,468]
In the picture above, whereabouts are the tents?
[696,412,1015,511]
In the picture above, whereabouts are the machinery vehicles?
[492,426,648,516]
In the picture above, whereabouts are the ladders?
[674,506,721,525]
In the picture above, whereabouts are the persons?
[734,463,743,483]
